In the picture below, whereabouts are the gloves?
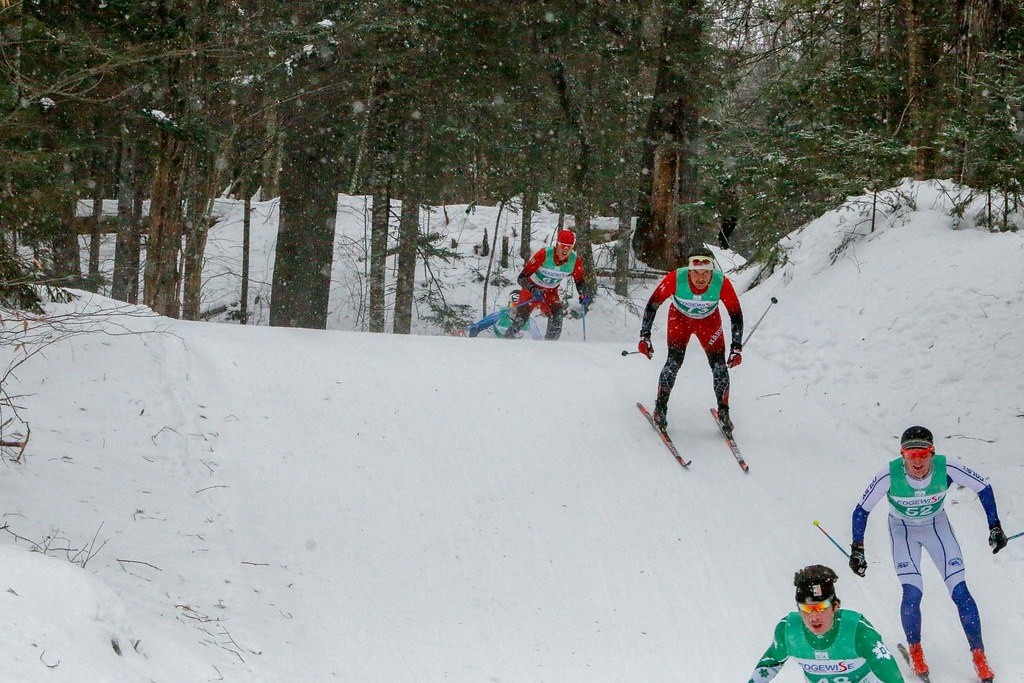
[579,295,591,305]
[531,288,544,302]
[849,545,868,577]
[638,330,654,359]
[988,523,1007,554]
[726,343,742,368]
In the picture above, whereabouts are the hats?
[900,425,935,455]
[557,229,574,247]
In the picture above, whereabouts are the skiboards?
[897,643,993,683]
[637,402,749,472]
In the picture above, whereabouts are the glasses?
[690,258,711,266]
[796,597,833,614]
[901,448,929,460]
[560,243,573,251]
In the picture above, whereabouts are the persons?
[719,184,738,250]
[748,566,905,683]
[638,247,744,435]
[849,426,1007,683]
[506,230,592,340]
[470,290,541,340]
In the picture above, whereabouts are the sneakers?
[972,649,994,681]
[718,408,734,431]
[653,399,667,426]
[907,643,929,676]
